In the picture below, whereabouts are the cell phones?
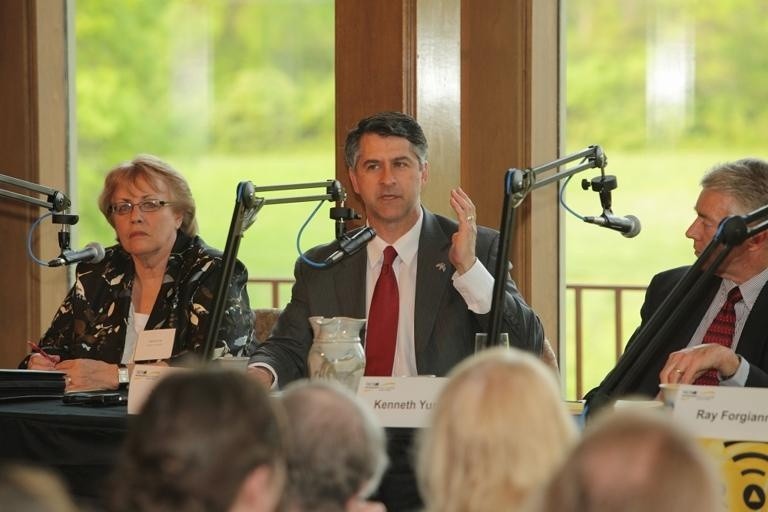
[63,390,122,406]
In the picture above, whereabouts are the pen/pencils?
[25,339,58,366]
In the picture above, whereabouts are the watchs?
[113,363,130,391]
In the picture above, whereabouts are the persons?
[403,346,579,511]
[582,158,768,407]
[243,109,544,393]
[13,152,253,394]
[86,357,296,511]
[272,377,393,512]
[543,408,727,512]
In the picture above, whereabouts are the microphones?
[585,213,641,238]
[324,225,377,267]
[46,241,106,269]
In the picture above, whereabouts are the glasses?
[108,198,178,215]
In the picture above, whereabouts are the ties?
[693,286,743,385]
[365,246,399,376]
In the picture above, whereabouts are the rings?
[466,216,473,222]
[674,368,685,376]
[66,375,72,384]
